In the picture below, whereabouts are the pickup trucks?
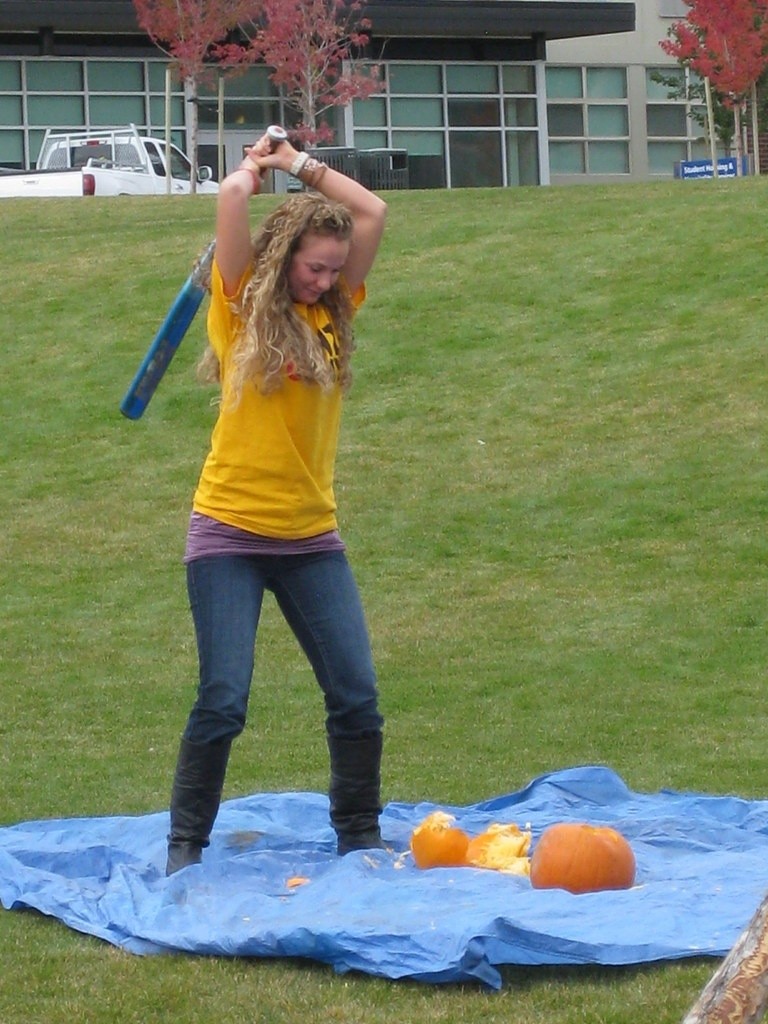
[0,124,222,197]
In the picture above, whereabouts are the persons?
[165,130,385,883]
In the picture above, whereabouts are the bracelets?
[236,167,259,194]
[289,151,327,188]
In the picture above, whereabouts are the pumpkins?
[530,820,636,895]
[411,810,532,869]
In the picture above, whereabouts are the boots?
[165,737,232,877]
[326,736,389,857]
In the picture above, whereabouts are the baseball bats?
[118,123,289,421]
